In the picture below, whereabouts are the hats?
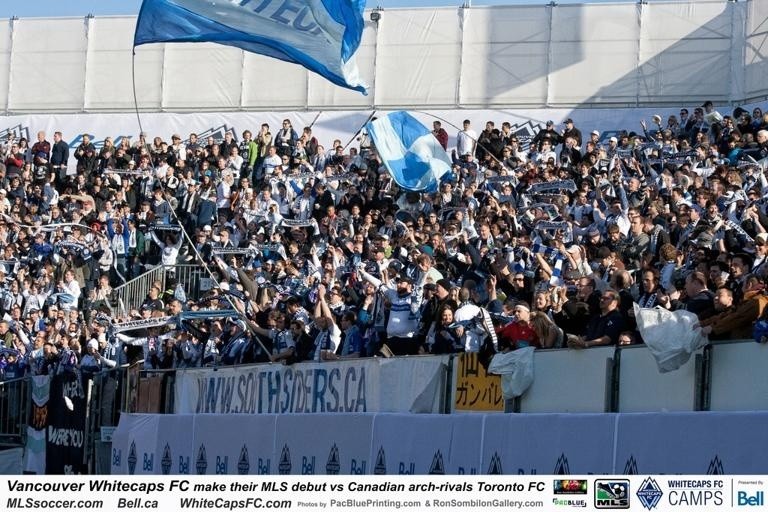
[0,100,768,351]
